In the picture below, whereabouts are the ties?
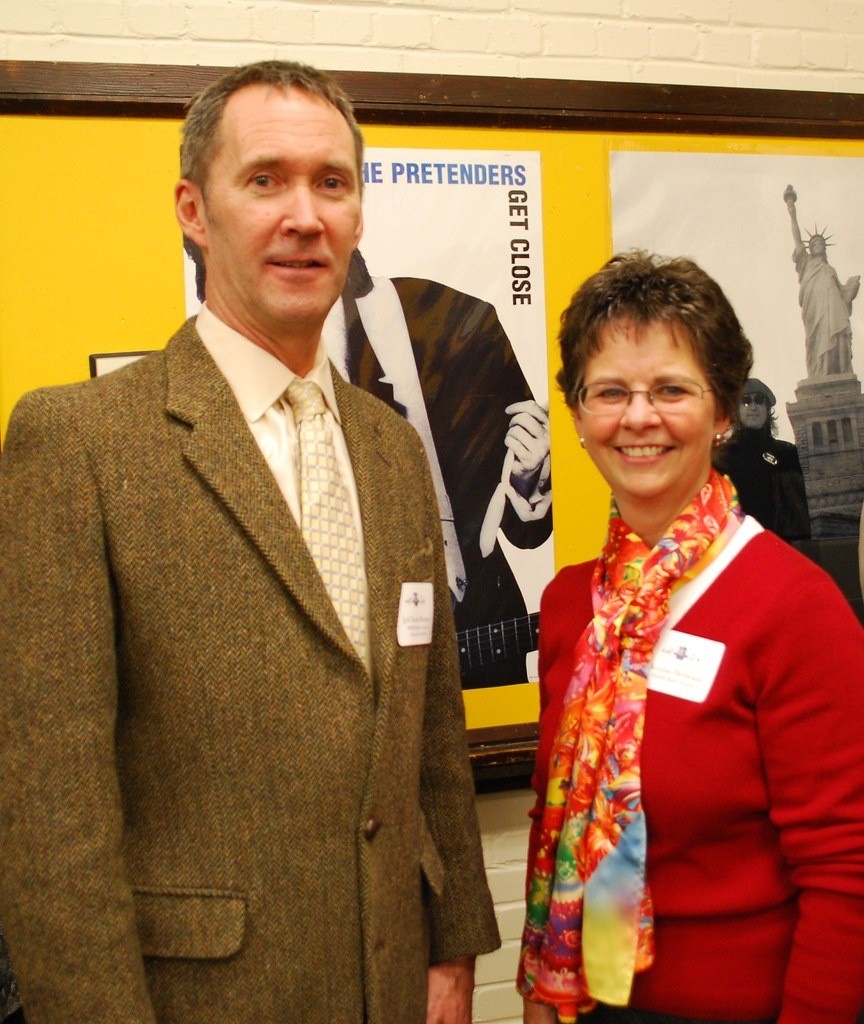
[283,379,372,676]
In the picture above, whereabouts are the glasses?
[741,392,766,405]
[576,379,715,414]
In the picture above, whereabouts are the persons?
[181,232,556,692]
[781,182,861,375]
[711,377,813,559]
[515,250,864,1023]
[0,62,504,1024]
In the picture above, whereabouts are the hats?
[741,378,776,406]
